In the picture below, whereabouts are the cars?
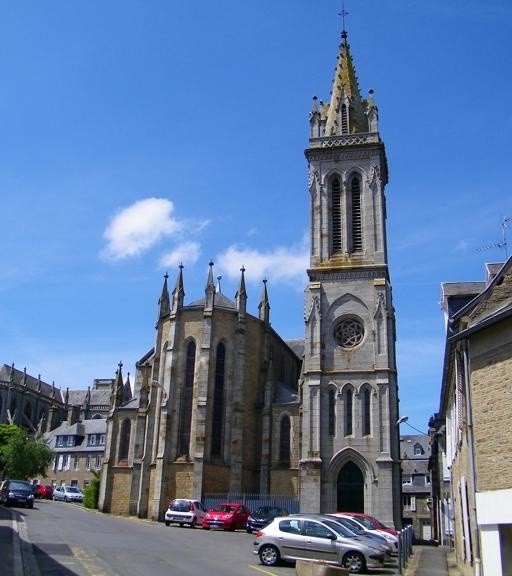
[164,497,398,574]
[0,480,83,508]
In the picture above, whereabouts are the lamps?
[395,416,408,426]
